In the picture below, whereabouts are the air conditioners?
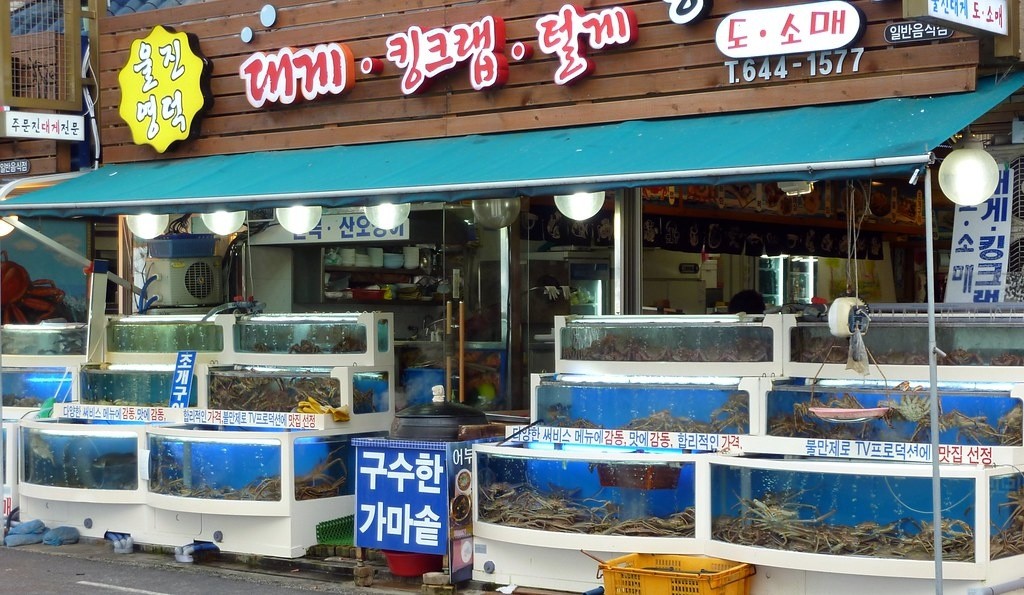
[143,255,222,307]
[124,213,173,241]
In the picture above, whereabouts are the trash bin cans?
[403,367,457,408]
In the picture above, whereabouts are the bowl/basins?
[421,296,433,301]
[380,550,443,577]
[325,291,342,299]
[339,247,419,269]
[351,289,385,304]
[451,468,472,522]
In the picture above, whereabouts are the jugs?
[420,248,433,269]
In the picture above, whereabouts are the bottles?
[344,287,354,302]
[789,262,808,298]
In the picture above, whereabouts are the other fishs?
[92,452,136,468]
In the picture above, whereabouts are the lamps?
[552,190,607,222]
[0,213,21,236]
[201,209,250,238]
[277,205,327,235]
[362,202,415,232]
[470,195,522,229]
[937,136,1002,214]
[778,180,814,197]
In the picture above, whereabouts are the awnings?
[0,68,1024,594]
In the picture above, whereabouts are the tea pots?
[323,247,342,266]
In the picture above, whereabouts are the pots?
[433,292,457,306]
[391,385,489,441]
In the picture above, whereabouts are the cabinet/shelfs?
[322,246,472,311]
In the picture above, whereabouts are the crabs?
[3,393,42,407]
[252,342,270,353]
[288,339,323,354]
[794,336,1024,366]
[151,444,348,501]
[329,337,366,354]
[30,433,55,467]
[209,375,375,412]
[563,333,771,362]
[478,381,1024,564]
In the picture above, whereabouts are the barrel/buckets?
[400,365,457,406]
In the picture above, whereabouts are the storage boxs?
[148,232,219,260]
[345,286,389,300]
[0,304,396,565]
[467,307,1024,595]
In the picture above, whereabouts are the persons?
[729,289,766,314]
